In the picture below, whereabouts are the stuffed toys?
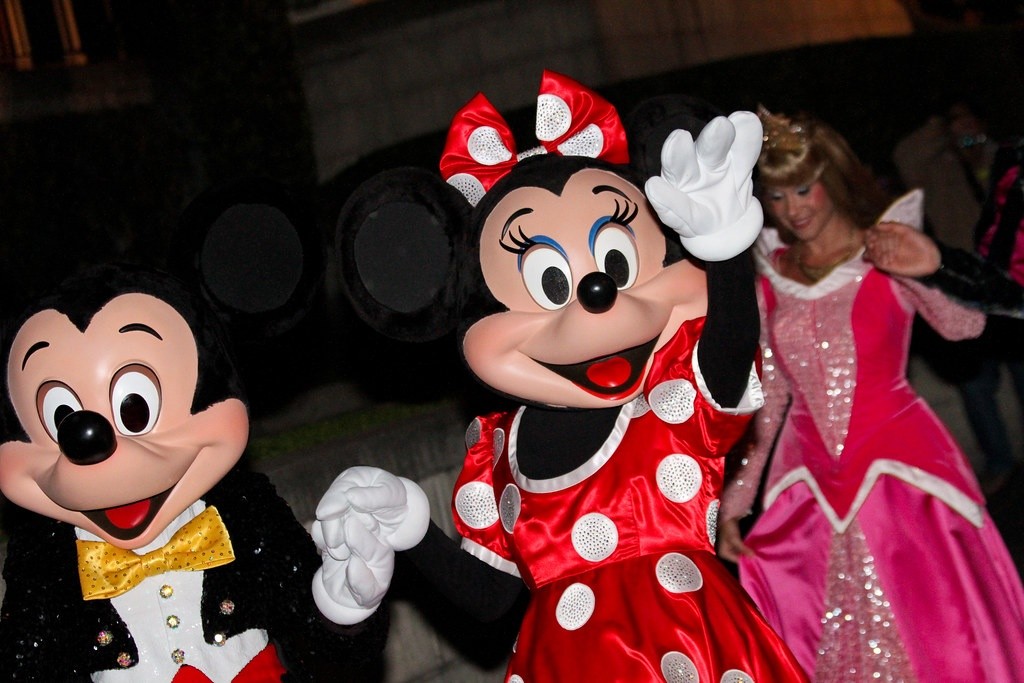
[0,196,396,683]
[313,69,806,683]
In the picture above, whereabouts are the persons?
[714,106,1024,683]
